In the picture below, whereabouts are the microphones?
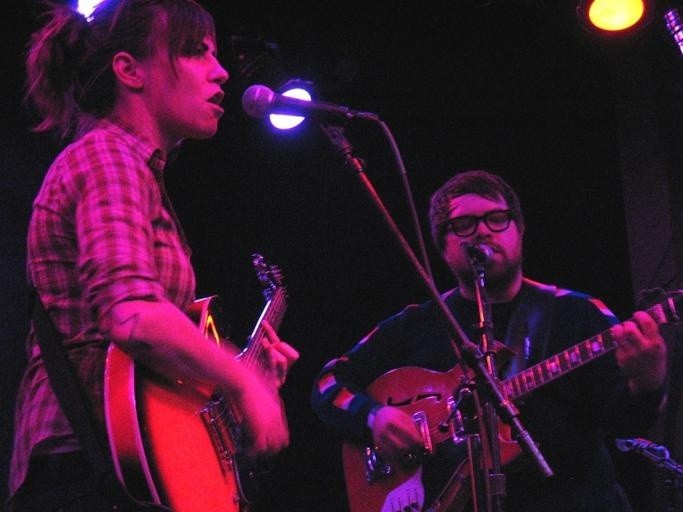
[463,242,494,267]
[241,84,349,126]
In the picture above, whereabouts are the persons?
[5,1,302,512]
[308,168,670,512]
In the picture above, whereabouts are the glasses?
[441,209,513,239]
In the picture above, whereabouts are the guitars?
[104,252,290,512]
[341,290,681,512]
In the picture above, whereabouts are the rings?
[620,340,629,346]
